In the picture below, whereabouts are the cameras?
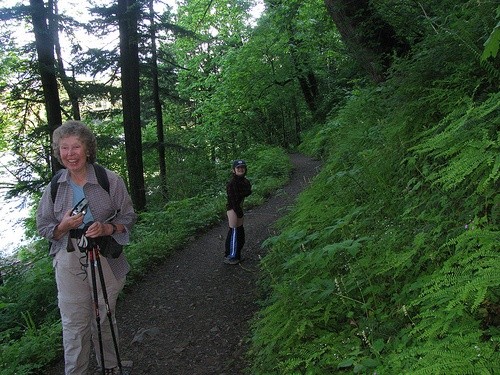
[72,198,89,217]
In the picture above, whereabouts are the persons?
[35,120,137,375]
[223,160,253,265]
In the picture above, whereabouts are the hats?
[233,160,246,168]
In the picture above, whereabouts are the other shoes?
[224,255,244,265]
[104,365,117,375]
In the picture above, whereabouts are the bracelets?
[106,222,117,236]
